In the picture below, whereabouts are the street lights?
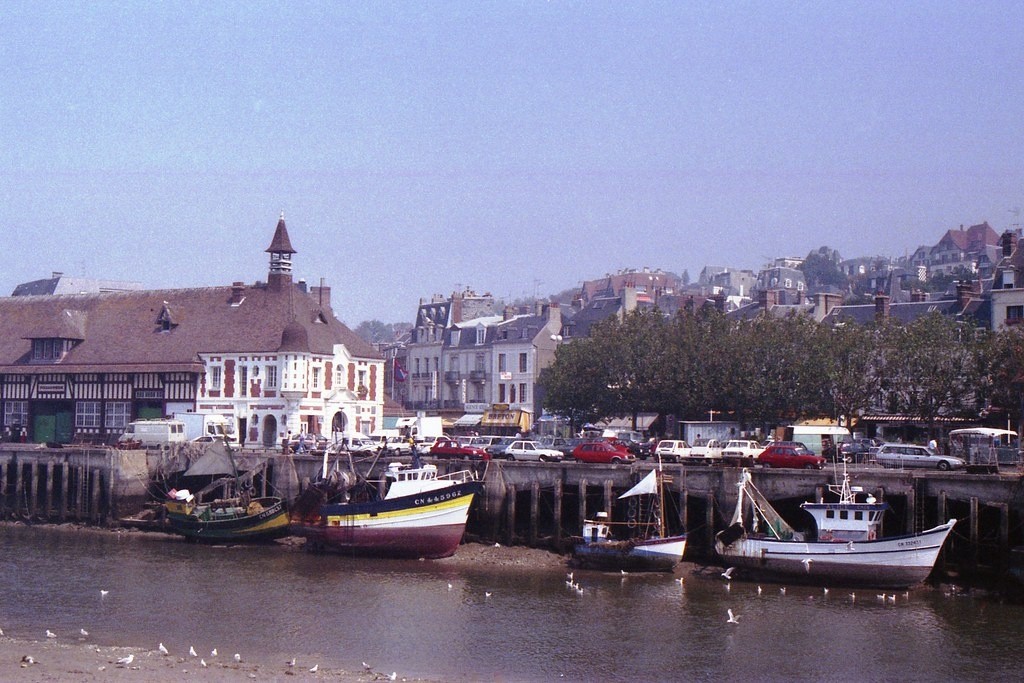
[339,406,344,438]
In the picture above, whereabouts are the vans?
[342,432,373,452]
[690,438,723,464]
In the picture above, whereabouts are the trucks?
[369,417,443,444]
[118,413,239,448]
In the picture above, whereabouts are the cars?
[430,435,649,463]
[767,440,815,456]
[573,442,636,464]
[758,445,826,470]
[822,438,884,465]
[189,434,241,450]
[282,434,327,451]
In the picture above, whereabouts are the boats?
[290,397,487,561]
[715,401,957,588]
[574,430,685,573]
[162,421,290,542]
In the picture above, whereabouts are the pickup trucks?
[876,442,965,470]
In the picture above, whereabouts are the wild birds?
[620,551,914,626]
[189,645,198,657]
[200,659,207,667]
[211,648,218,658]
[563,571,586,594]
[99,589,108,596]
[80,628,90,636]
[45,629,58,639]
[384,672,396,681]
[495,543,500,548]
[486,592,492,597]
[116,654,136,666]
[233,654,242,663]
[447,582,453,591]
[282,657,297,674]
[21,655,35,666]
[158,642,168,654]
[844,540,855,551]
[360,661,374,671]
[306,664,319,673]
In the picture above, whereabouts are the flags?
[394,358,408,383]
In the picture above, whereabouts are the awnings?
[452,414,484,428]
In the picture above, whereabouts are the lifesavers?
[653,509,662,518]
[245,477,253,488]
[653,496,661,507]
[653,519,662,530]
[653,484,661,495]
[629,497,637,507]
[627,508,637,518]
[627,518,636,528]
[869,531,876,541]
[651,530,661,538]
[249,487,257,497]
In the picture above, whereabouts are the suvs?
[654,440,692,462]
[386,436,411,456]
[721,440,765,463]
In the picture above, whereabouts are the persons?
[515,430,522,449]
[750,431,772,443]
[287,428,307,454]
[694,433,703,446]
[929,437,941,455]
